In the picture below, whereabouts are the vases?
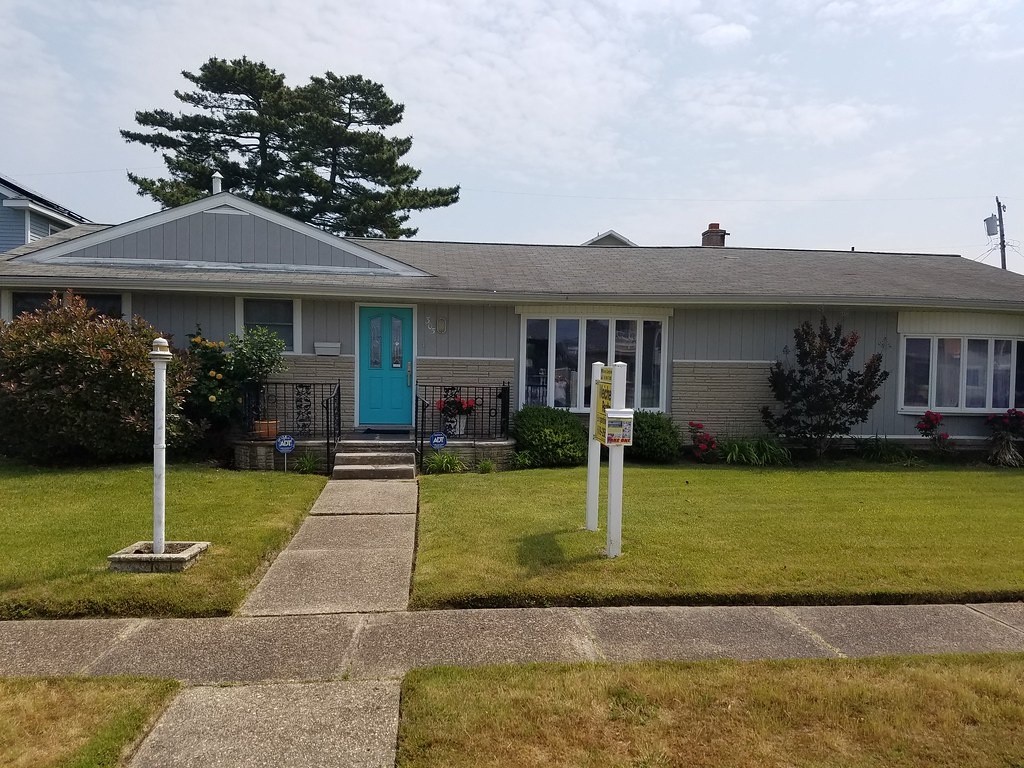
[255,419,280,437]
[444,415,466,434]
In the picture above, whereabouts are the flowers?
[689,422,715,458]
[435,394,475,414]
[915,411,950,440]
[190,336,226,402]
[986,409,1024,431]
